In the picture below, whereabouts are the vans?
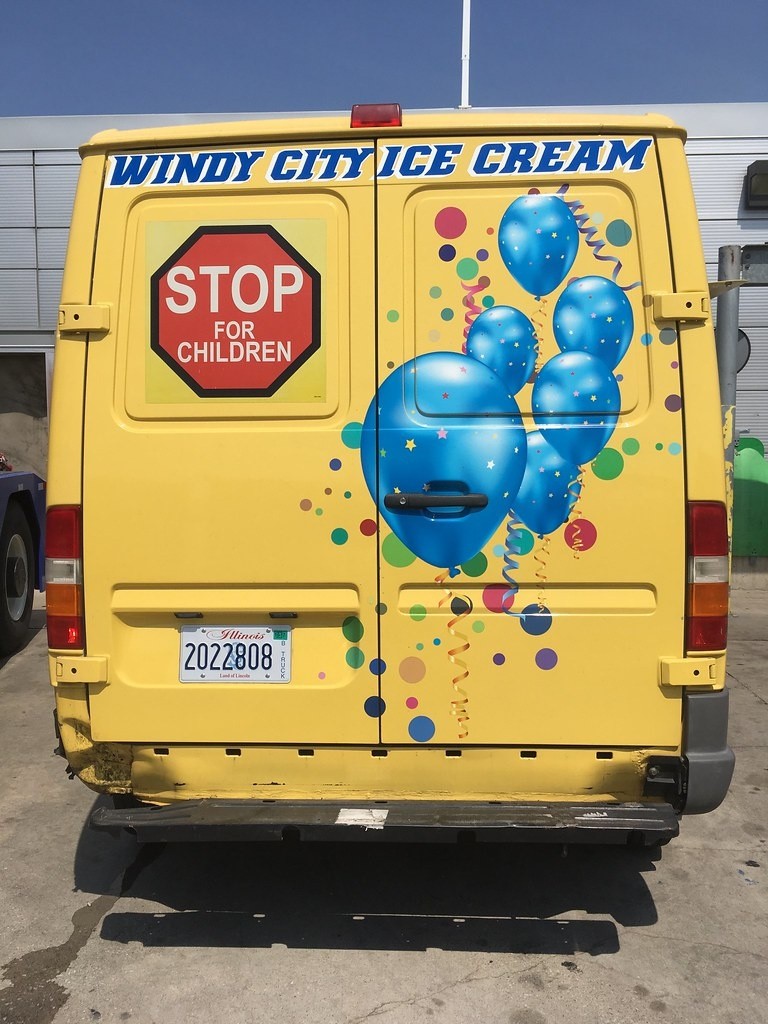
[45,103,751,851]
